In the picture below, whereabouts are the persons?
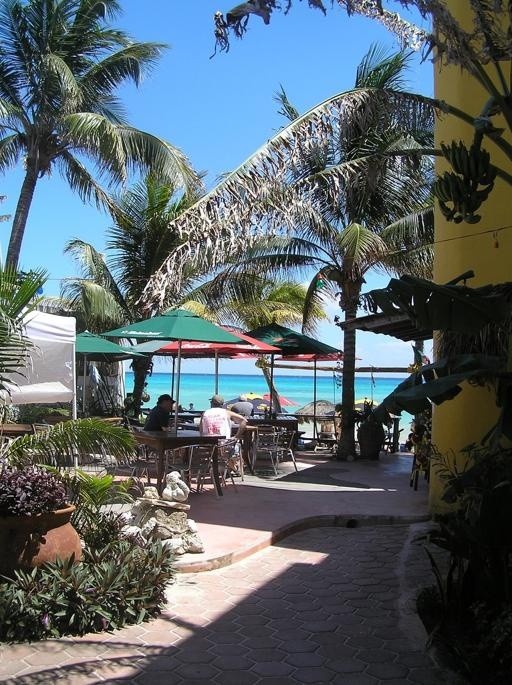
[404,433,415,453]
[198,394,249,476]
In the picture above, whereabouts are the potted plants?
[0,464,82,577]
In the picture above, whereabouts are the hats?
[157,393,176,405]
[239,393,247,402]
[208,394,224,405]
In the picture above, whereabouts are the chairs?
[120,408,339,500]
